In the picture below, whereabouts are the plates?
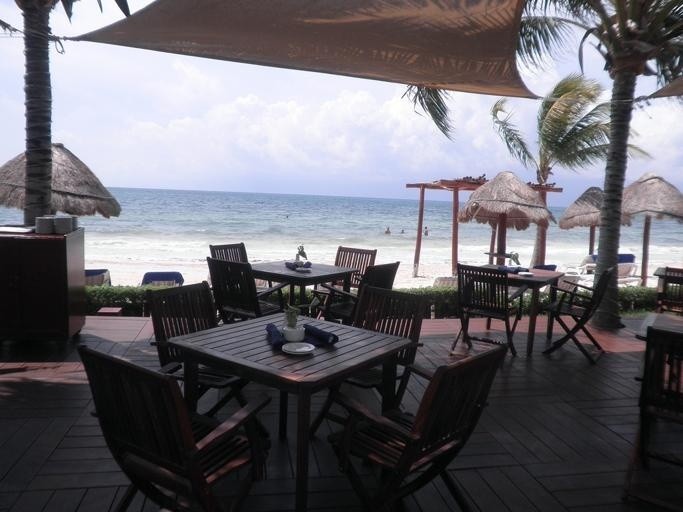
[517,272,532,276]
[281,342,315,354]
[295,267,311,272]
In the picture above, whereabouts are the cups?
[33,214,78,234]
